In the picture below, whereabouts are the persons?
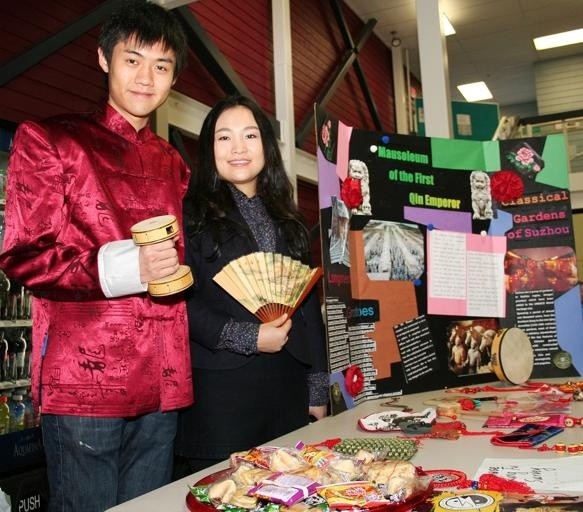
[182,94,330,468]
[449,335,466,375]
[463,338,482,374]
[0,0,195,512]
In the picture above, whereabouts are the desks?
[104,373,580,512]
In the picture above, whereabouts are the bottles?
[0,287,42,433]
